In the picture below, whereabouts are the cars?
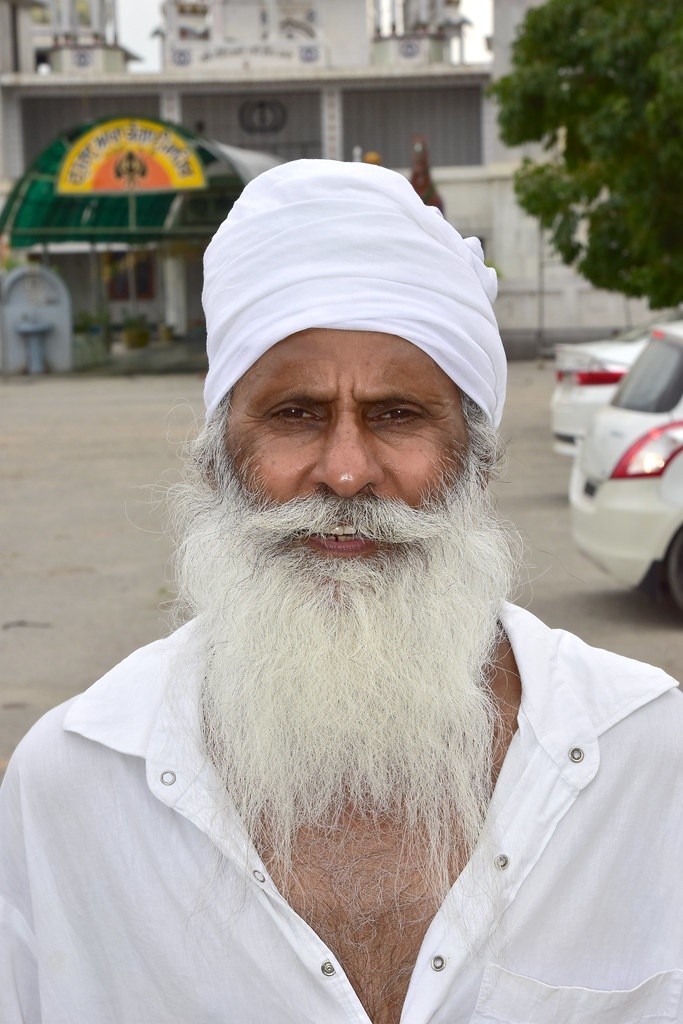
[566,318,683,619]
[547,320,671,458]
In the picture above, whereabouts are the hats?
[203,157,509,426]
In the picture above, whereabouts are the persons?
[0,157,682,1023]
